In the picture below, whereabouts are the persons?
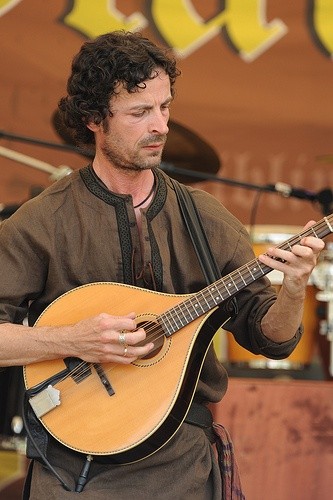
[0,30,324,500]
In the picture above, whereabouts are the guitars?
[20,212,333,468]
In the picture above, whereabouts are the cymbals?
[50,99,222,187]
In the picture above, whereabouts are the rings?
[123,345,129,358]
[119,333,127,344]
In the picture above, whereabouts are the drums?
[219,229,321,372]
[310,241,333,380]
